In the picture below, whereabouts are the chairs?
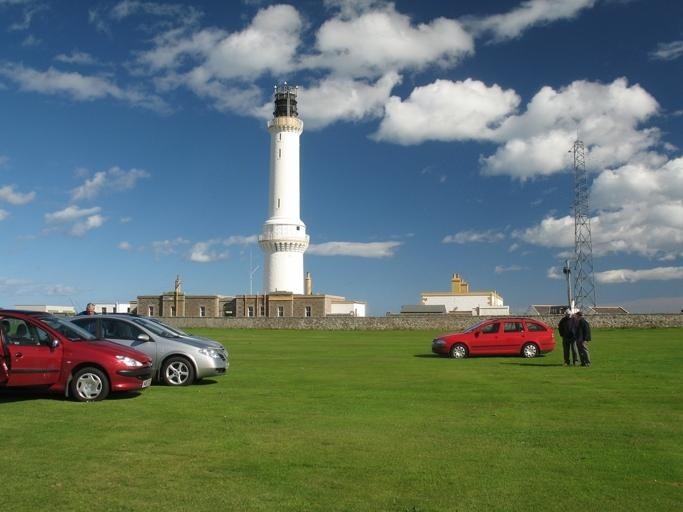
[2,320,29,345]
[492,325,497,332]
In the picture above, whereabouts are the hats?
[575,312,584,316]
[565,309,573,317]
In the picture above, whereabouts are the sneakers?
[563,361,591,367]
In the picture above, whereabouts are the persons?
[575,311,591,367]
[558,308,579,366]
[76,303,98,332]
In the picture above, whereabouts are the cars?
[432,317,555,360]
[24,312,230,386]
[0,310,153,402]
